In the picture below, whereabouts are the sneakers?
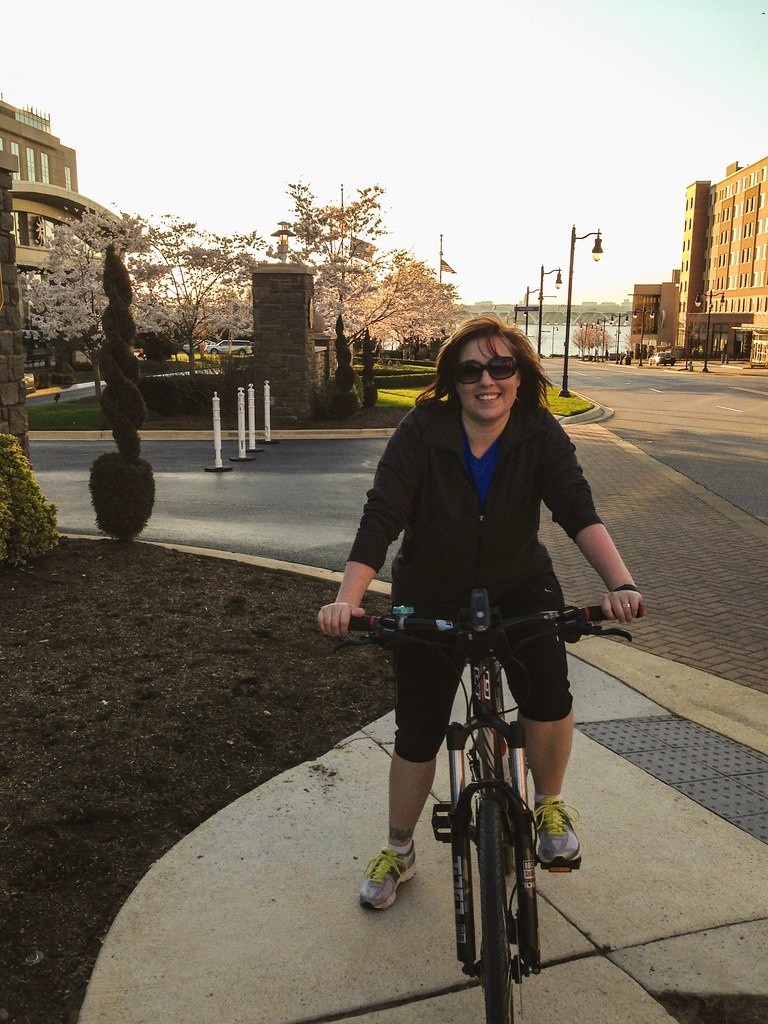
[532,796,583,864]
[359,840,416,910]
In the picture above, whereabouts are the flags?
[441,259,457,274]
[339,216,351,232]
[349,236,375,263]
[329,230,347,238]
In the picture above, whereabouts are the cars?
[649,351,675,367]
[204,339,252,357]
[183,341,216,353]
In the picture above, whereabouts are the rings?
[622,603,630,608]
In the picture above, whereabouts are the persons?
[137,348,145,360]
[316,315,645,911]
[412,345,423,355]
[375,339,382,358]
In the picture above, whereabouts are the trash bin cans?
[625,357,631,365]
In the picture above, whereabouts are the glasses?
[451,356,519,385]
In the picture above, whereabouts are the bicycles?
[325,602,646,1024]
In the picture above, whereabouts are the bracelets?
[614,584,642,594]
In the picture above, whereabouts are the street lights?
[694,289,725,373]
[632,307,656,368]
[525,286,544,337]
[580,323,594,362]
[558,225,603,399]
[536,265,562,359]
[596,318,613,363]
[610,313,629,364]
